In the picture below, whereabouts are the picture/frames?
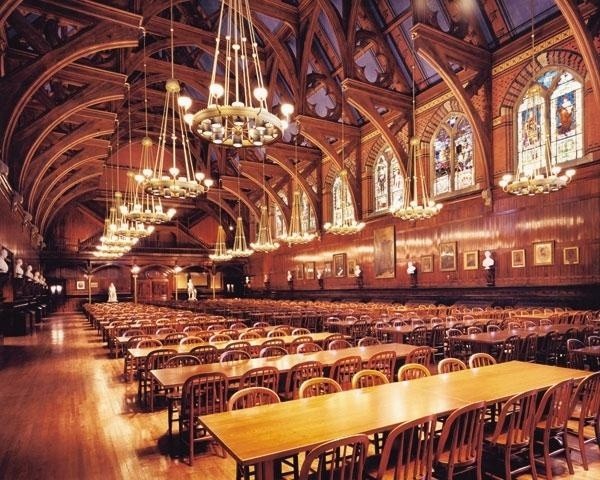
[294,251,359,280]
[419,240,581,274]
[75,279,100,291]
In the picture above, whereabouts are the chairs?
[3,294,48,337]
[82,296,584,478]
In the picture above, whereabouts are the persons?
[337,266,344,276]
[481,251,495,270]
[352,265,363,278]
[316,269,323,280]
[106,281,119,303]
[263,274,268,282]
[286,270,293,281]
[0,248,49,290]
[379,234,395,276]
[406,261,417,275]
[245,276,250,284]
[186,278,198,301]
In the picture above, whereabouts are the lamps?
[183,0,295,151]
[387,2,445,225]
[226,151,254,260]
[207,192,230,263]
[277,152,317,248]
[320,2,369,237]
[90,0,215,260]
[497,0,578,199]
[250,151,279,255]
